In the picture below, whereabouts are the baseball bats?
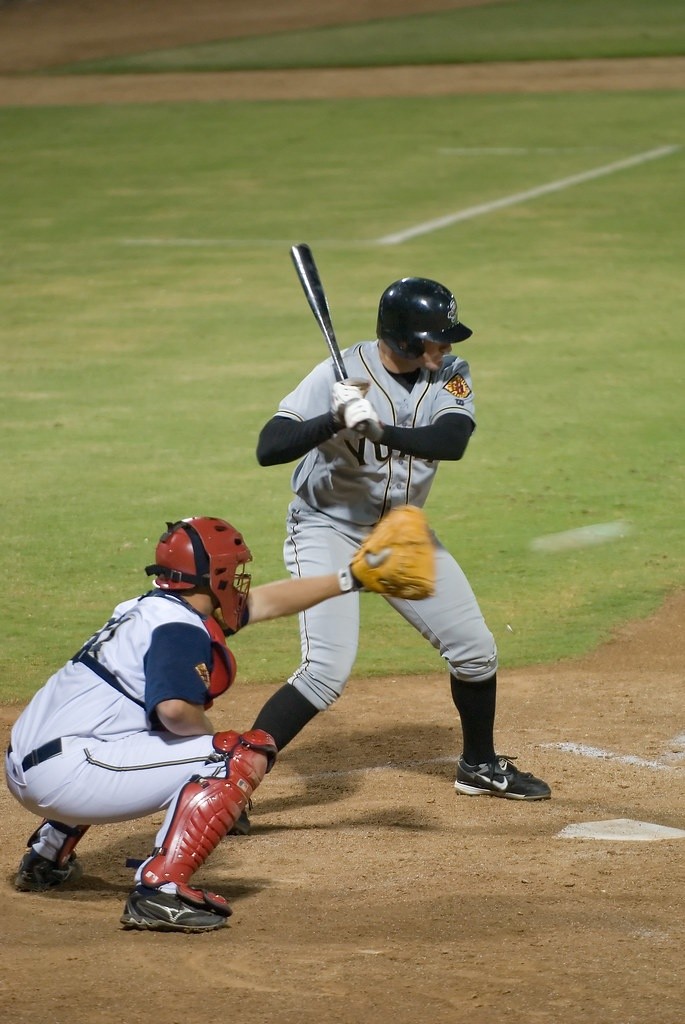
[287,240,368,434]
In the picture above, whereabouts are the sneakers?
[229,809,251,836]
[120,888,228,934]
[453,754,552,801]
[14,849,82,892]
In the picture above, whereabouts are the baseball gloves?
[337,503,438,601]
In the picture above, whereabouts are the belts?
[7,738,63,777]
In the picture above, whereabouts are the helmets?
[376,276,473,360]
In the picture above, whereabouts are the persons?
[231,276,551,835]
[4,516,391,932]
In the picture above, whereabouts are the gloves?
[329,374,383,444]
